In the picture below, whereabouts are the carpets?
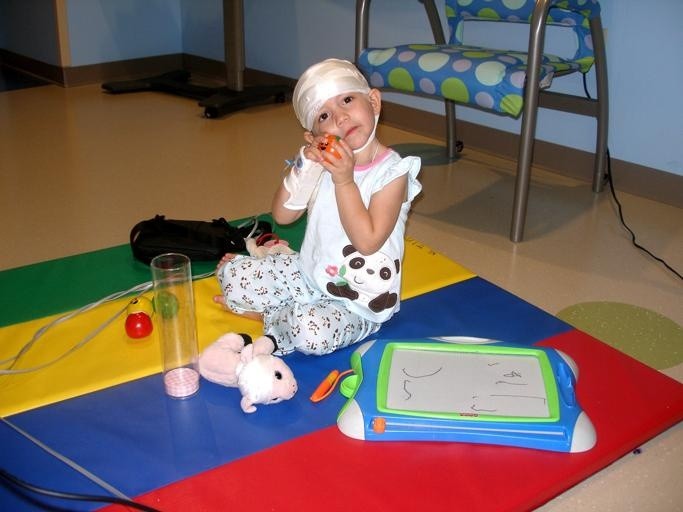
[0,203,683,512]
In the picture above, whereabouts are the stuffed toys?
[198,332,298,414]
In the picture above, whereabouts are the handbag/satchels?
[130,214,273,267]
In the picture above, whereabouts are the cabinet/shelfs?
[352,0,610,242]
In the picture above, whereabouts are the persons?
[210,58,423,357]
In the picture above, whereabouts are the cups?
[151,253,200,400]
